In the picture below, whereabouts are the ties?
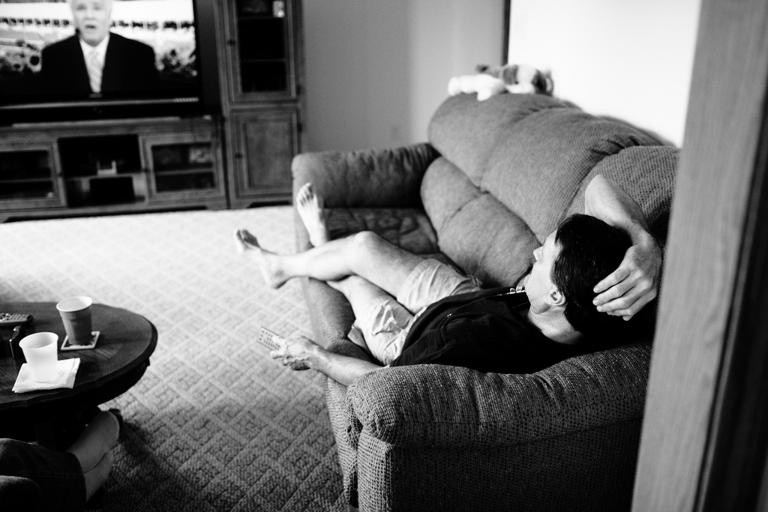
[86,47,102,94]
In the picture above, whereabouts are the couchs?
[290,92,683,512]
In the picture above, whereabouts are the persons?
[40,0,156,100]
[232,171,663,386]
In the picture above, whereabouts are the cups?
[19,296,96,382]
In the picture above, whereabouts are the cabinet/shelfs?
[216,1,308,211]
[0,115,227,223]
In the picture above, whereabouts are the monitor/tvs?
[0,0,219,123]
